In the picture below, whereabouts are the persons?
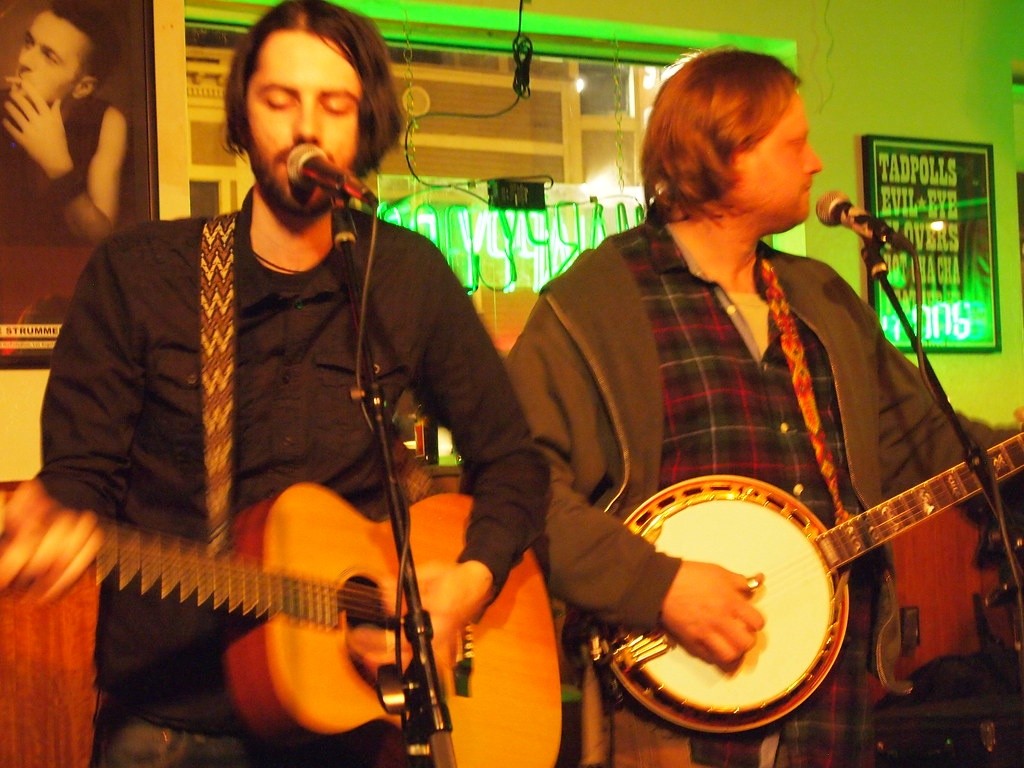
[2,0,555,768]
[478,45,1023,766]
[1,0,134,248]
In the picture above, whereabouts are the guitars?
[585,426,1024,736]
[1,481,564,768]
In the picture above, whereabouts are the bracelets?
[48,168,85,207]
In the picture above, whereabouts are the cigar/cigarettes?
[4,76,24,85]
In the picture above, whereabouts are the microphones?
[285,143,379,206]
[816,191,890,246]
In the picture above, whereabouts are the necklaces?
[243,233,342,278]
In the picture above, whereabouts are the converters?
[483,180,548,209]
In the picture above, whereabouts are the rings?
[746,575,759,591]
